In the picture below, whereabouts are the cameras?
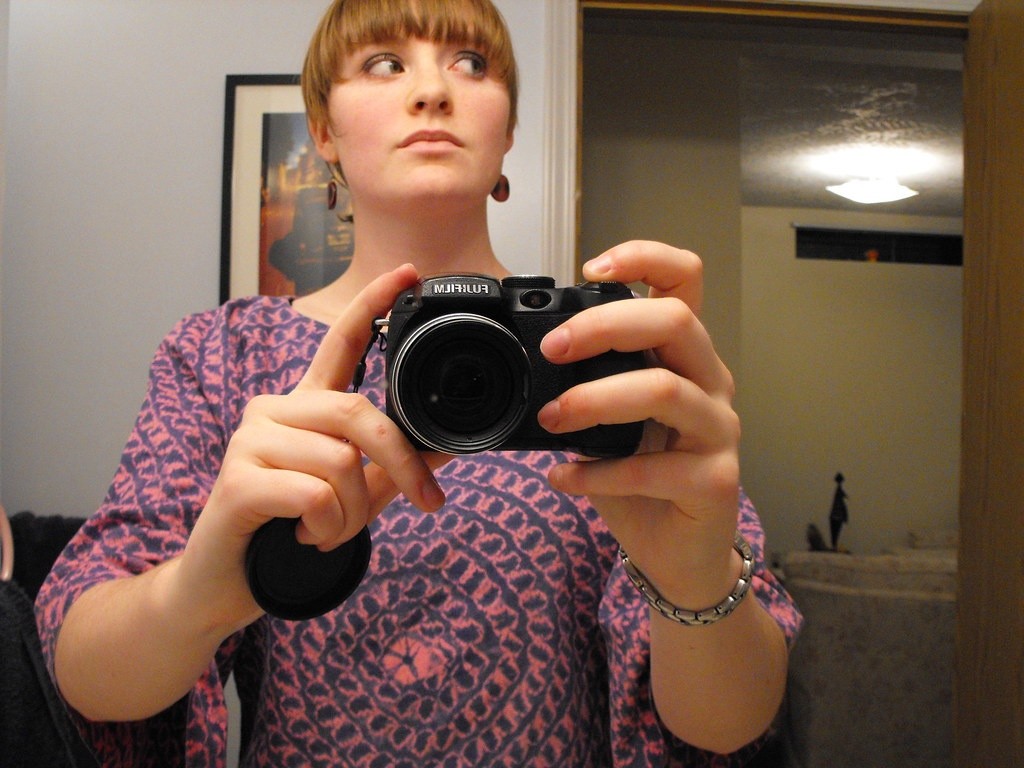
[385,275,644,460]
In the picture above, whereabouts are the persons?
[32,0,804,768]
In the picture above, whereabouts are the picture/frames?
[220,74,355,304]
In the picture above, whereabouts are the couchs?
[775,528,956,768]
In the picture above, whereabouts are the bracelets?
[619,529,755,625]
